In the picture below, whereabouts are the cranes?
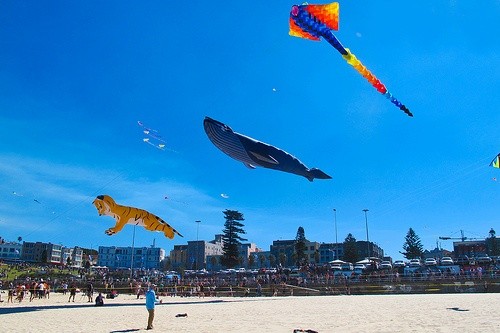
[438,230,487,242]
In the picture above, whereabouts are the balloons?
[91,195,184,239]
[203,117,334,183]
[284,3,417,117]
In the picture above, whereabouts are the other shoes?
[148,326,152,329]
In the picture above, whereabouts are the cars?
[409,259,420,268]
[184,265,303,276]
[394,260,405,268]
[425,258,437,266]
[330,262,379,271]
[439,257,454,266]
[453,254,500,266]
[380,261,392,270]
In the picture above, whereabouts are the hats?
[151,284,158,287]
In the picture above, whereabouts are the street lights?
[195,220,201,270]
[363,209,371,263]
[333,208,338,259]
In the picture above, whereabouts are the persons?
[146,284,163,329]
[68,287,75,303]
[87,284,94,302]
[95,292,105,306]
[1,262,500,303]
